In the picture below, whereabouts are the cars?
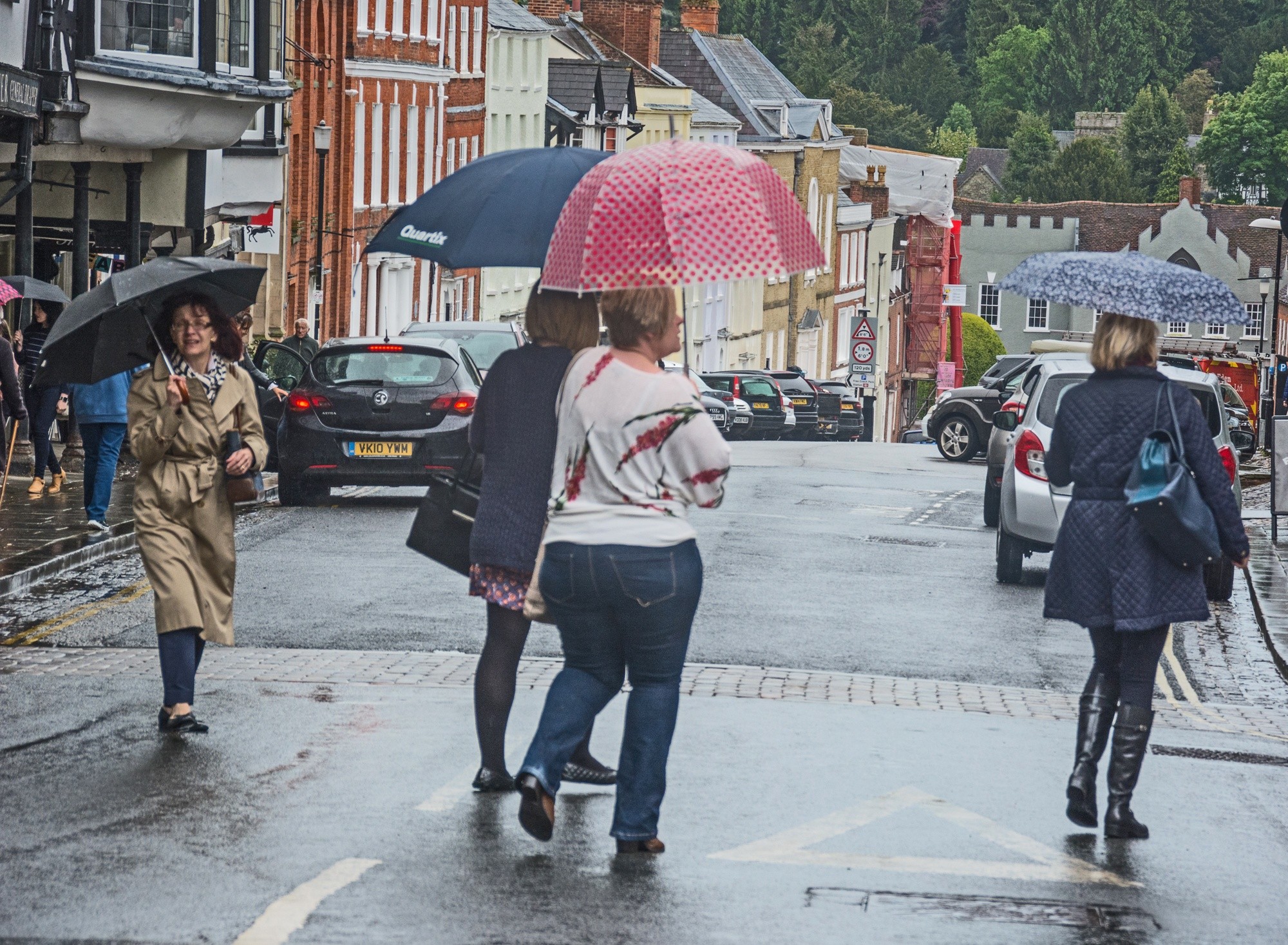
[655,360,796,441]
[763,370,864,442]
[983,352,1255,601]
[901,335,1260,461]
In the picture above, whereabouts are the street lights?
[1249,216,1283,452]
[1256,273,1271,449]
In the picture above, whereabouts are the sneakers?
[87,519,110,529]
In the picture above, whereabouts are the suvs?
[252,321,529,505]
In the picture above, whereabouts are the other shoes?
[472,767,516,790]
[158,707,208,731]
[562,762,617,785]
[167,711,196,728]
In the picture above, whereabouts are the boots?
[1102,703,1156,839]
[1065,677,1120,828]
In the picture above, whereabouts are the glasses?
[172,317,213,331]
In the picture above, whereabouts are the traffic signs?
[845,374,875,389]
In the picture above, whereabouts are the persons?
[275,317,320,390]
[1040,312,1252,837]
[515,267,731,850]
[467,268,619,791]
[227,309,291,402]
[0,305,28,488]
[14,299,67,493]
[63,370,130,533]
[132,300,273,739]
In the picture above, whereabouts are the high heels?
[48,466,67,492]
[514,773,554,842]
[27,477,46,494]
[615,837,665,853]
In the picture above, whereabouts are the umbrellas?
[0,276,70,344]
[0,278,23,306]
[995,248,1253,326]
[357,137,616,269]
[534,141,827,375]
[38,253,269,405]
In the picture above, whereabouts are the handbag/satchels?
[523,517,559,625]
[225,428,258,503]
[406,446,481,579]
[1124,379,1221,566]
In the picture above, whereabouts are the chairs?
[338,359,366,380]
[419,358,442,379]
